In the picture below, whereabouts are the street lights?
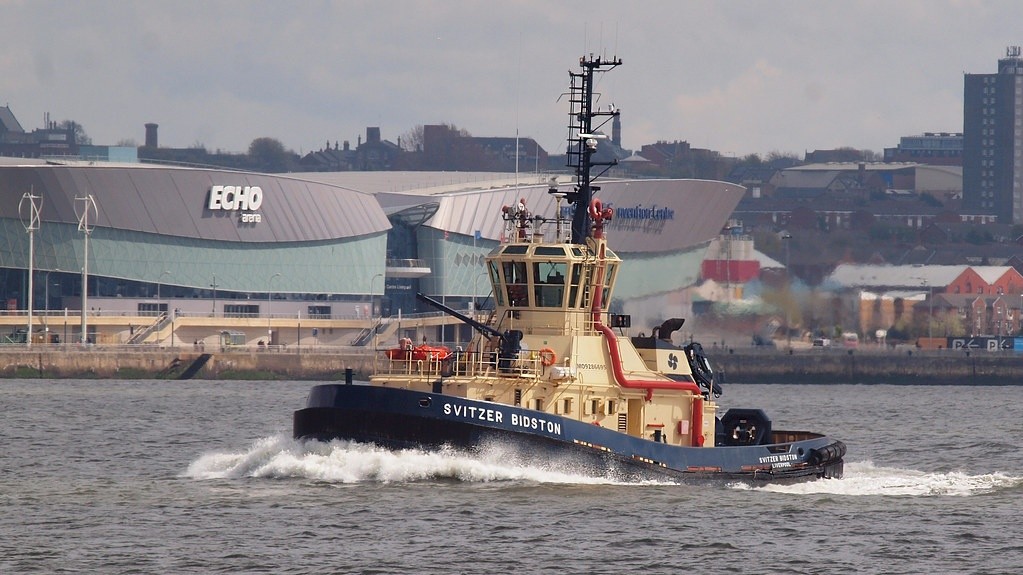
[269,272,281,347]
[369,272,383,346]
[156,270,170,342]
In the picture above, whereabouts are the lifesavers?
[539,349,555,367]
[591,420,600,426]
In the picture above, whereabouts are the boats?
[285,51,850,489]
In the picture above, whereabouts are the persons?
[553,272,564,284]
[509,278,526,299]
